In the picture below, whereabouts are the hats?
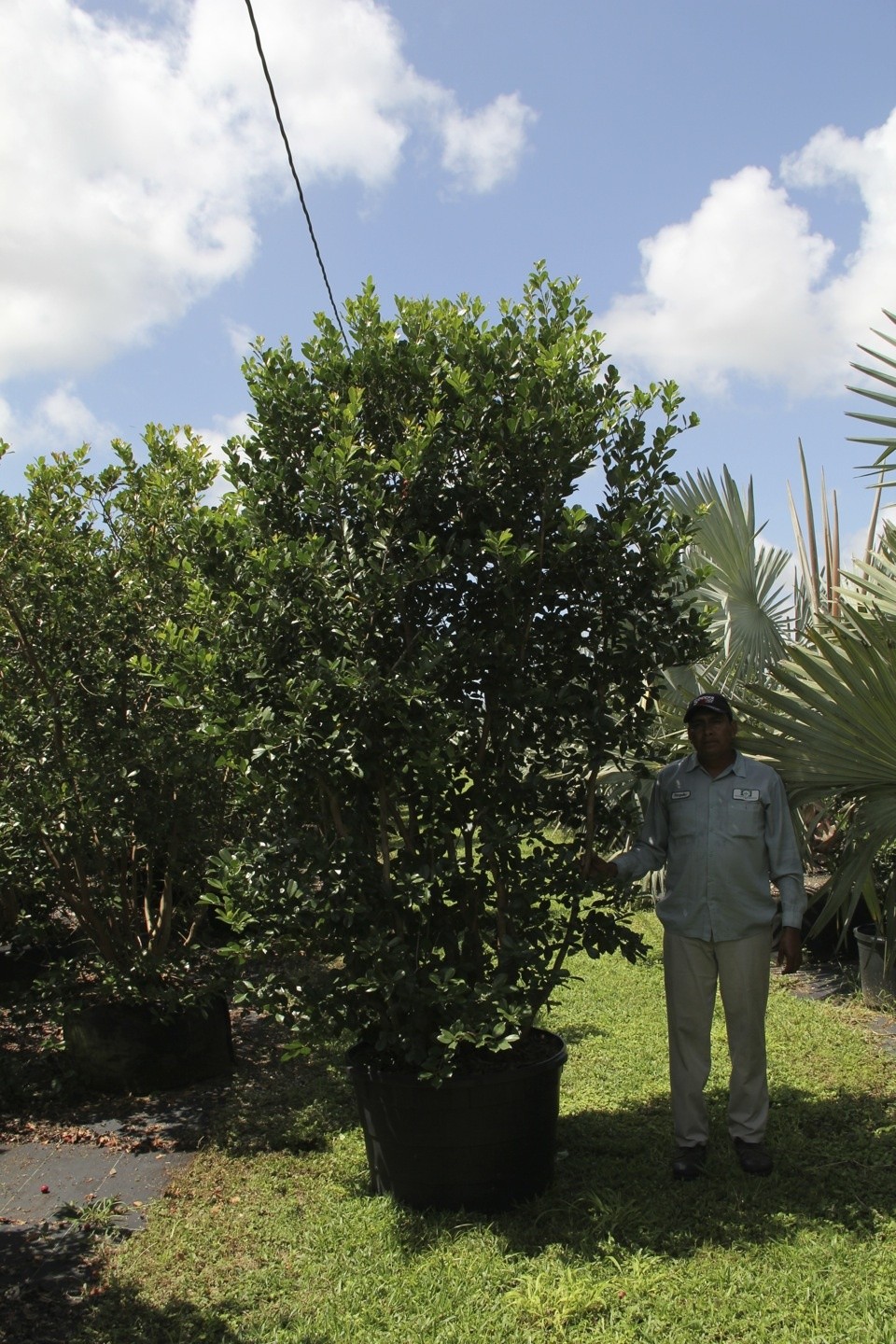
[684,693,733,724]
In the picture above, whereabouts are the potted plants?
[0,259,896,1218]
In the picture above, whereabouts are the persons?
[580,692,808,1181]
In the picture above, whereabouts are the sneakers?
[736,1136,772,1175]
[672,1146,705,1178]
